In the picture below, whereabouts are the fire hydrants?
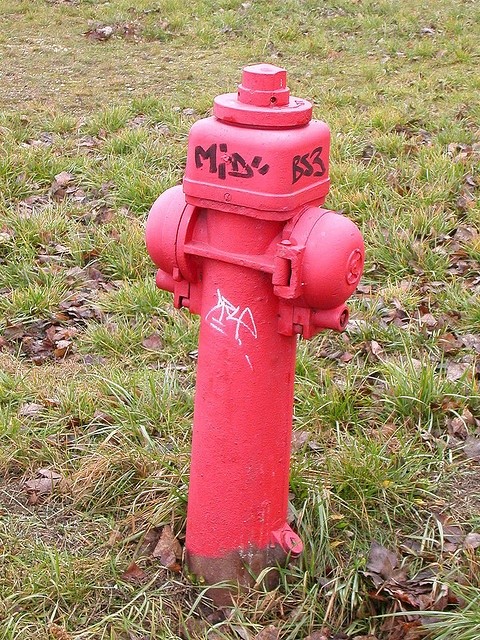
[145,63,365,617]
